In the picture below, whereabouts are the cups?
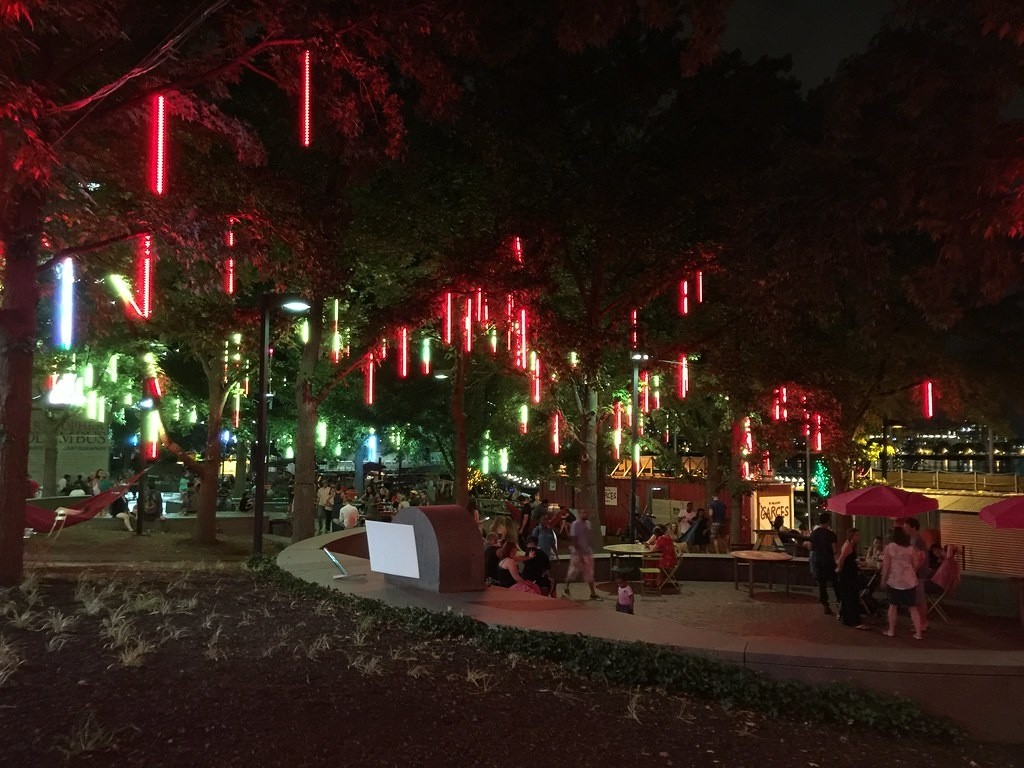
[866,557,874,567]
[638,543,642,548]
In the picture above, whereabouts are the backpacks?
[144,491,158,515]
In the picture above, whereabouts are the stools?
[640,567,662,595]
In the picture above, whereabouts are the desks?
[603,543,669,592]
[730,551,792,599]
[857,561,930,616]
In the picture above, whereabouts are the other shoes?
[824,606,832,613]
[317,530,323,536]
[883,631,896,637]
[590,594,603,600]
[914,633,924,640]
[854,624,869,629]
[565,588,571,597]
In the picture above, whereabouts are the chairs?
[926,555,959,622]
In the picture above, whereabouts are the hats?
[527,535,538,543]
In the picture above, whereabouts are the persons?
[615,575,635,615]
[27,468,604,602]
[644,491,733,590]
[771,515,960,640]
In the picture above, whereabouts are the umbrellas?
[827,484,939,545]
[979,496,1024,529]
